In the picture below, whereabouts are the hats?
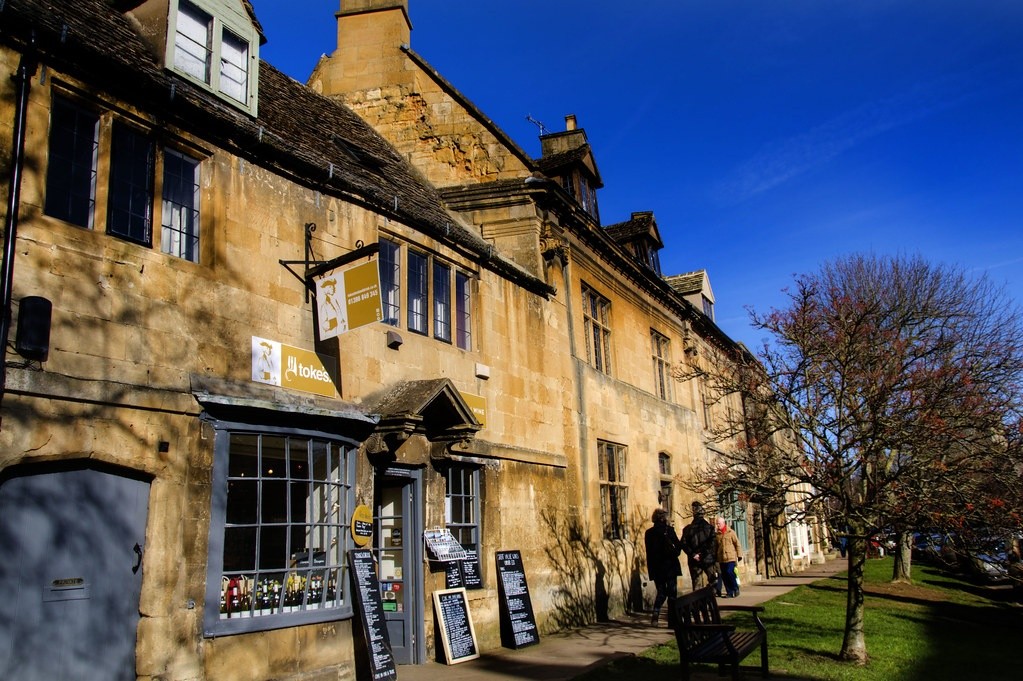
[692,501,705,516]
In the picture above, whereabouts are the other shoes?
[721,594,733,598]
[734,588,740,597]
[651,620,658,628]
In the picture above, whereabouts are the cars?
[884,533,897,553]
[912,530,1023,596]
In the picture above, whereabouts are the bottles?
[220,572,344,620]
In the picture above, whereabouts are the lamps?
[658,484,672,503]
[386,331,403,348]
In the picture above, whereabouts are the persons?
[838,531,847,560]
[715,517,743,598]
[681,501,718,597]
[644,508,682,627]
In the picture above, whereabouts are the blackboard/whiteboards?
[495,549,540,651]
[433,587,480,665]
[446,561,464,589]
[460,544,483,589]
[347,549,398,681]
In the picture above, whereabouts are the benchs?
[671,586,768,681]
